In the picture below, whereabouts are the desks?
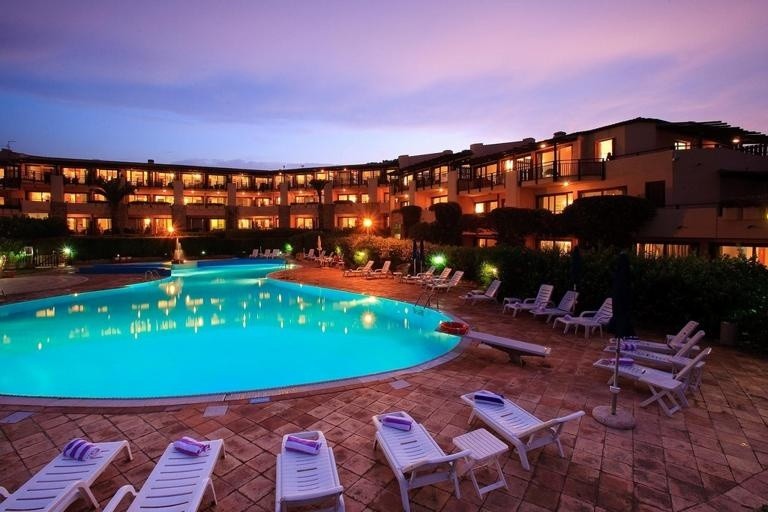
[450,427,511,499]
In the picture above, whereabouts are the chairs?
[592,320,713,419]
[0,428,134,512]
[502,284,554,317]
[248,246,502,305]
[458,387,586,472]
[530,291,578,324]
[99,435,226,512]
[271,429,347,512]
[552,298,613,341]
[368,410,479,512]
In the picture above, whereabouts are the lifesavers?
[441,321,469,335]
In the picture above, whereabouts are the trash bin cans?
[720,311,737,346]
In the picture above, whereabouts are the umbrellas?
[418,236,424,273]
[316,235,322,257]
[411,238,416,276]
[592,249,632,429]
[566,245,585,315]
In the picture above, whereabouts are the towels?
[172,435,210,458]
[62,437,102,462]
[284,431,321,456]
[377,412,412,431]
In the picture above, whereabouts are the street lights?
[168,226,174,237]
[363,296,374,329]
[166,278,177,297]
[363,216,374,237]
